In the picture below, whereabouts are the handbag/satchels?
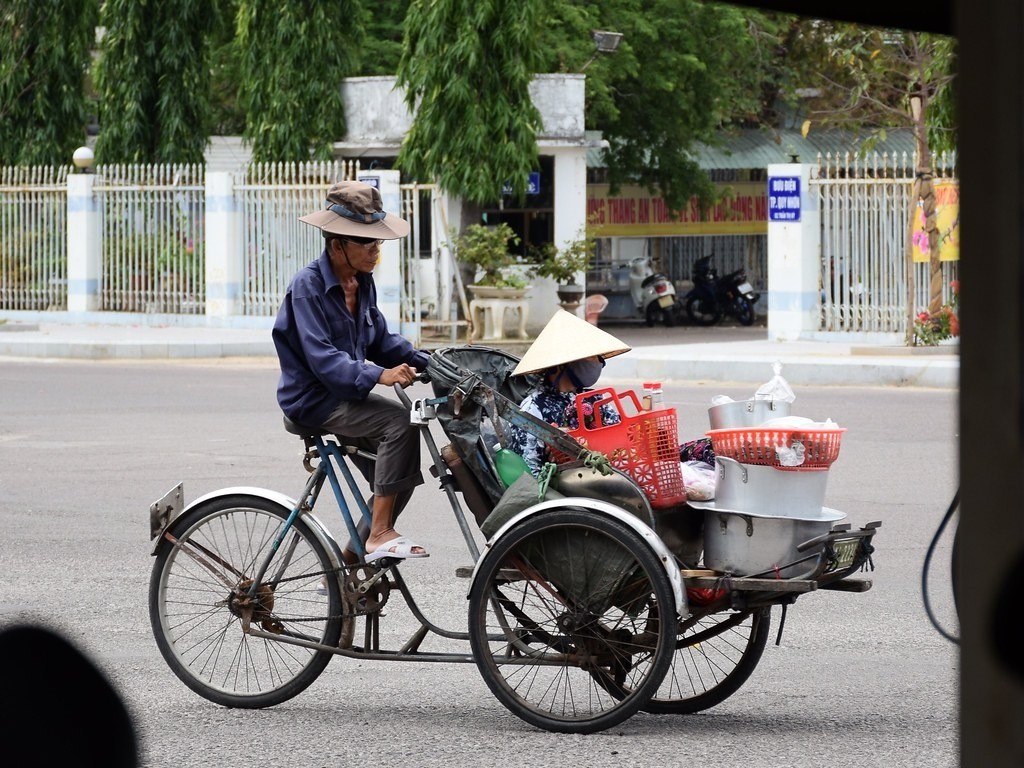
[547,387,686,508]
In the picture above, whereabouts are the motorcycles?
[682,254,761,325]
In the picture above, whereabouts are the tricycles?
[148,344,874,732]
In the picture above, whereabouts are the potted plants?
[525,224,604,304]
[440,222,533,298]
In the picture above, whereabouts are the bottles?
[492,442,537,495]
[641,382,671,457]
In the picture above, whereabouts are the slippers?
[317,582,387,617]
[363,537,430,564]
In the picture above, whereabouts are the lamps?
[73,147,95,173]
[580,27,624,73]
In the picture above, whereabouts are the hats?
[297,181,410,241]
[509,308,633,377]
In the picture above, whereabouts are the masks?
[562,358,603,387]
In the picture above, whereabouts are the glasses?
[339,237,385,251]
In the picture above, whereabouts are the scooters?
[619,254,678,326]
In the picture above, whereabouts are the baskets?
[707,424,846,471]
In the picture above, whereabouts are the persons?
[272,180,437,565]
[510,309,713,478]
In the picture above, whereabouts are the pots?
[705,391,792,433]
[681,455,847,580]
